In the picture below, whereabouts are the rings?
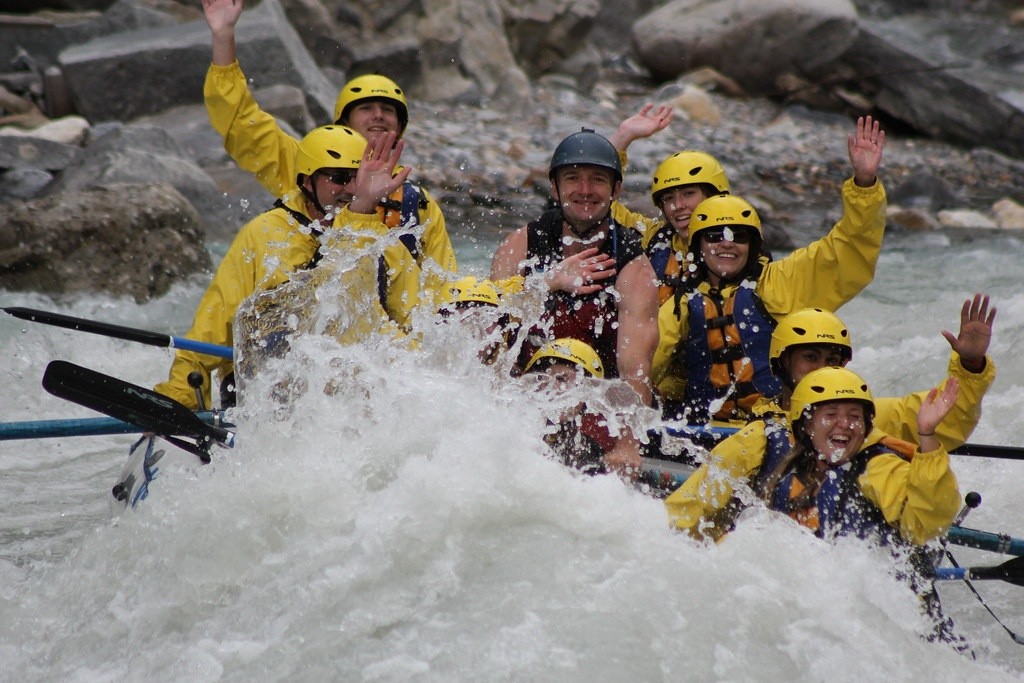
[871,140,877,144]
[944,398,947,402]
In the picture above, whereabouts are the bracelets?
[918,432,936,436]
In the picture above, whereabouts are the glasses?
[316,169,357,186]
[701,227,754,244]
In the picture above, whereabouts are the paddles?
[41,359,235,448]
[638,458,1024,557]
[0,305,233,358]
[0,407,235,440]
[935,556,1024,587]
[649,423,1024,460]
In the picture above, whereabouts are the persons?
[488,127,660,484]
[652,116,887,460]
[663,293,997,535]
[610,104,733,416]
[153,0,616,418]
[521,338,608,479]
[762,367,963,640]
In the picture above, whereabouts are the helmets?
[523,337,605,384]
[651,150,730,209]
[294,124,374,187]
[437,277,501,316]
[687,194,763,263]
[548,127,622,186]
[333,75,410,150]
[788,366,878,444]
[769,307,852,380]
